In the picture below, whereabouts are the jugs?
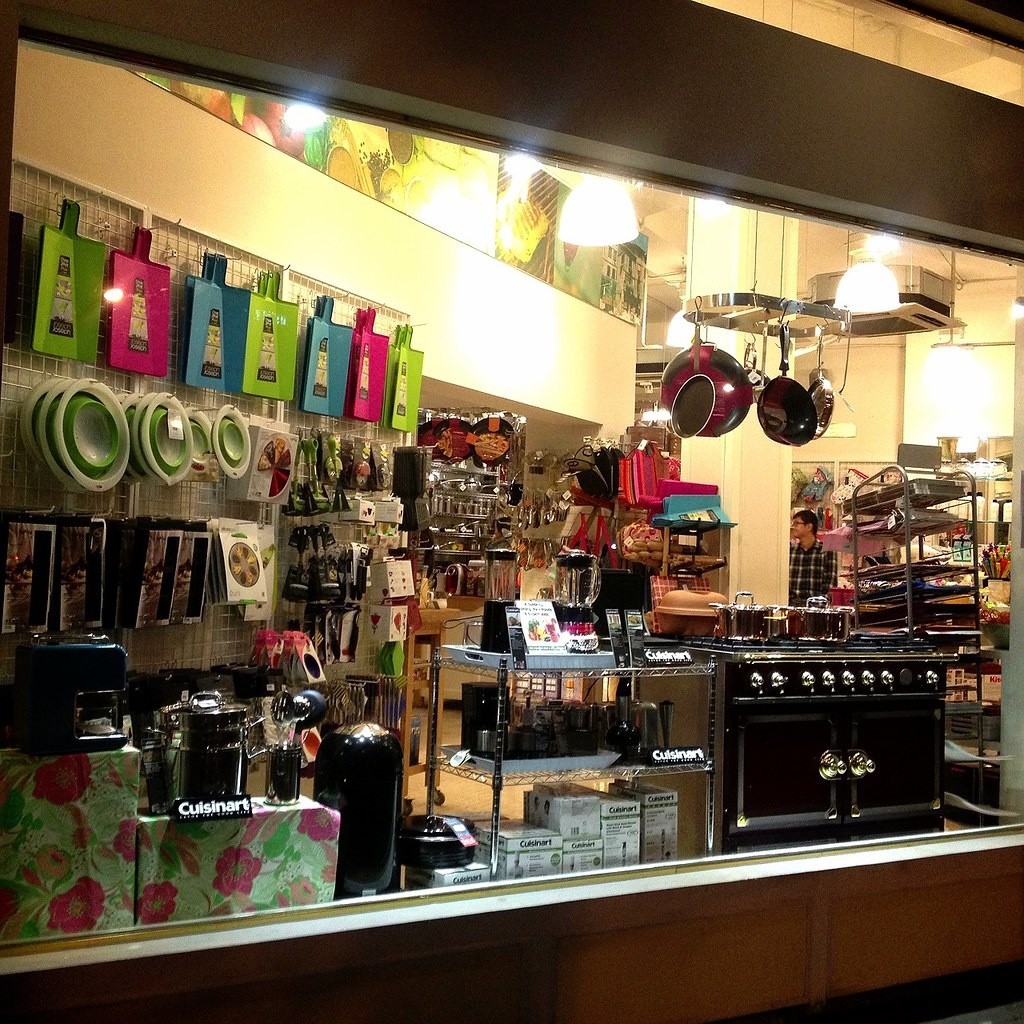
[556,549,601,623]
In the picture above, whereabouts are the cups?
[264,745,302,806]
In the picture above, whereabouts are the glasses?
[793,520,805,526]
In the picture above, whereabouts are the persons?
[788,509,838,635]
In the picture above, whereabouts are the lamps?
[555,170,904,348]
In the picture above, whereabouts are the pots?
[780,596,853,642]
[709,591,777,639]
[418,414,514,468]
[660,323,834,448]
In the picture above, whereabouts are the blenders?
[480,548,517,653]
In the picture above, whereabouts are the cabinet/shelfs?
[426,642,716,882]
[428,462,503,553]
[728,712,939,835]
[842,461,985,831]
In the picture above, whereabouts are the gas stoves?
[679,635,948,699]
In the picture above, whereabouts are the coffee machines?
[459,680,511,758]
[13,631,129,756]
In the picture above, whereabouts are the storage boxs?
[655,589,728,636]
[472,776,678,878]
[0,413,412,644]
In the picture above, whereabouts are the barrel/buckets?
[179,690,249,795]
